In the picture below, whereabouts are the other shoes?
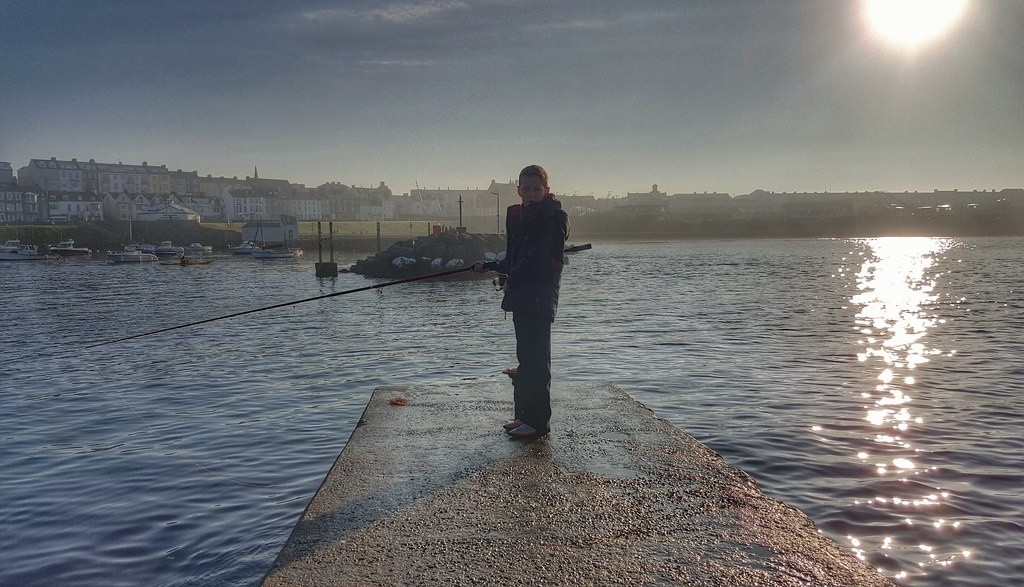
[509,418,551,438]
[502,419,525,430]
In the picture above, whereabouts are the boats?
[107,250,158,263]
[106,212,214,254]
[50,238,92,257]
[227,213,266,253]
[250,231,296,259]
[0,231,49,260]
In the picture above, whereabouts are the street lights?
[490,192,500,237]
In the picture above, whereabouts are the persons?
[500,165,571,440]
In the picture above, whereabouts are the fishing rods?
[83,241,593,351]
